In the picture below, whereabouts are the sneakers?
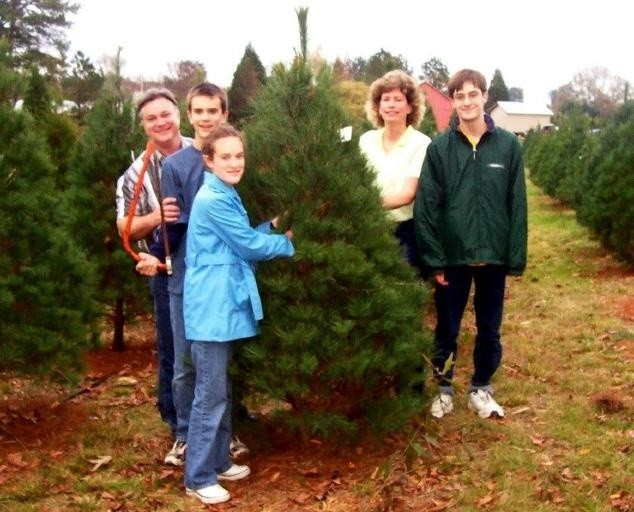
[164,436,250,504]
[430,389,504,419]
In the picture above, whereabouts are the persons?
[134,81,251,468]
[115,88,193,430]
[357,69,431,266]
[413,68,528,417]
[181,129,295,505]
